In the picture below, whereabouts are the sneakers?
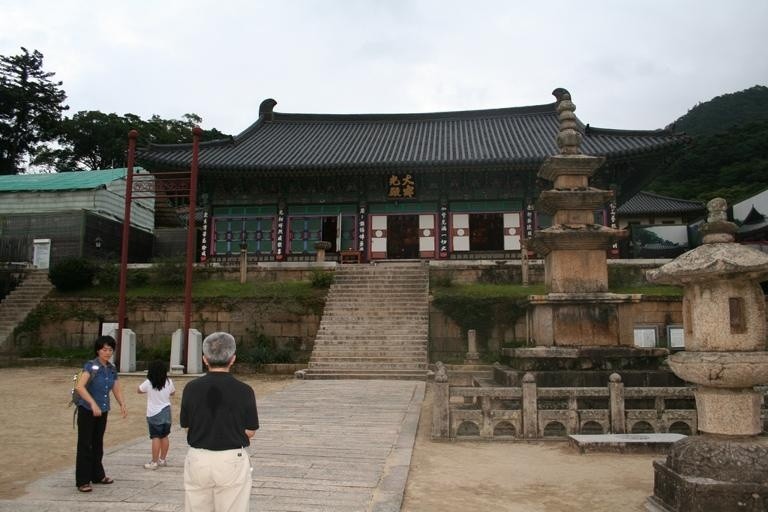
[144,462,158,469]
[158,458,167,466]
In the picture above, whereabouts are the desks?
[338,249,363,264]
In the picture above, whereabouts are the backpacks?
[69,360,116,407]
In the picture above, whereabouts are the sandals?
[78,484,92,492]
[101,477,114,484]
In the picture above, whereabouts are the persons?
[179,330,259,512]
[135,357,177,470]
[69,334,128,492]
